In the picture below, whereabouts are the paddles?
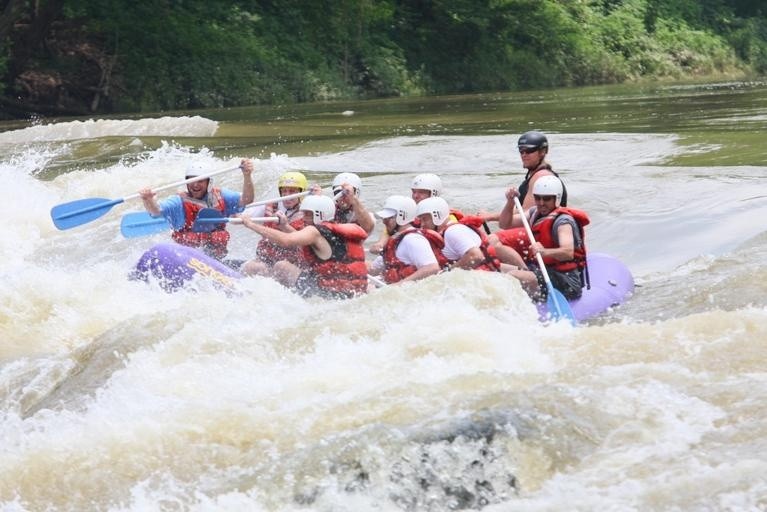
[513,195,578,328]
[120,211,171,239]
[51,164,243,231]
[191,207,280,233]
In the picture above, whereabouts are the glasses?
[534,194,556,202]
[519,147,542,154]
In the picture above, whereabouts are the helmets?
[519,132,548,149]
[185,162,215,194]
[533,175,563,208]
[411,173,442,197]
[300,195,335,225]
[417,197,450,226]
[332,173,362,198]
[374,195,418,226]
[278,171,308,203]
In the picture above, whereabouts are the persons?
[475,129,568,270]
[136,158,256,263]
[498,175,591,303]
[229,157,505,305]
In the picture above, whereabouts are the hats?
[376,209,395,221]
[332,187,342,193]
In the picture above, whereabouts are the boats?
[129,241,636,327]
